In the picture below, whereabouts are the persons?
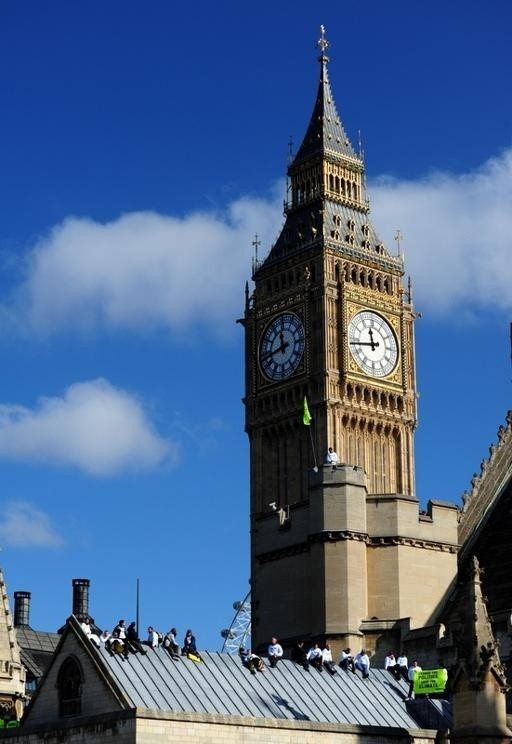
[326,445,340,465]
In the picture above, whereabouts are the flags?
[302,394,312,426]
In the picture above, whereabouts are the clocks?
[348,310,402,379]
[256,310,307,383]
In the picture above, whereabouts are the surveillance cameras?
[269,502,277,511]
